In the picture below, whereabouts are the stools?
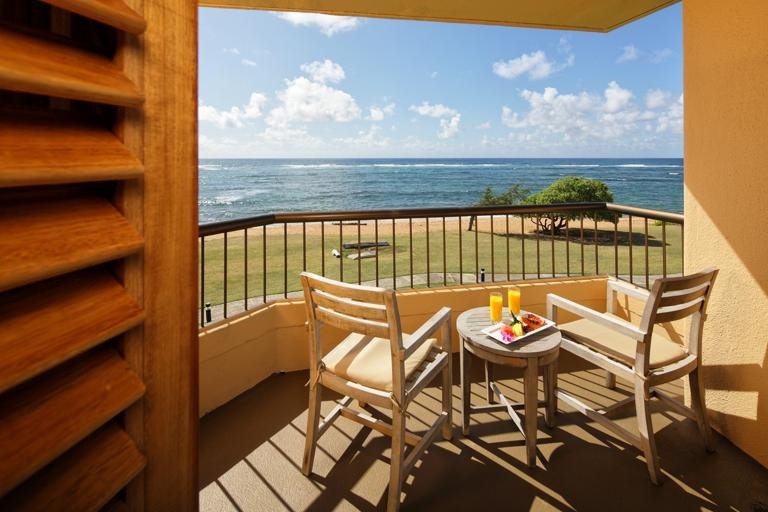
[456,306,562,469]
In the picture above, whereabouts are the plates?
[480,312,556,345]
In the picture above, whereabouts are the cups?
[489,292,504,324]
[508,286,521,316]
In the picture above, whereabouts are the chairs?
[299,272,452,512]
[546,266,719,486]
